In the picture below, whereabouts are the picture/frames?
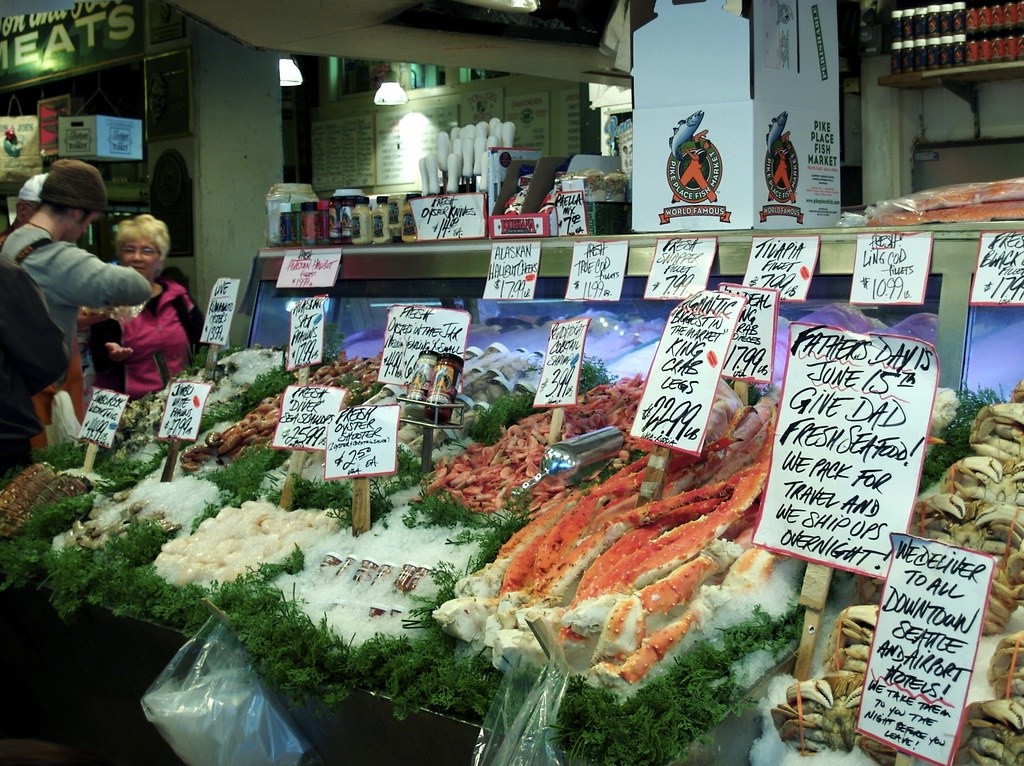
[37,95,71,156]
[143,46,195,142]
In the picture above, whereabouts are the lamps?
[374,62,408,105]
[399,109,431,131]
[278,54,303,86]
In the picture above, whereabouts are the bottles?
[425,353,464,423]
[404,350,439,419]
[890,2,967,74]
[262,183,422,244]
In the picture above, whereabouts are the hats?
[17,172,49,202]
[40,160,108,213]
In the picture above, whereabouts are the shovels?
[511,427,626,505]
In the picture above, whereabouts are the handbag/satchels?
[0,95,43,182]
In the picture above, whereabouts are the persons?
[89,215,205,404]
[0,256,69,481]
[0,159,151,447]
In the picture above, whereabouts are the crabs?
[432,378,1024,766]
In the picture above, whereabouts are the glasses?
[122,246,159,257]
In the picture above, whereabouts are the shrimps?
[179,351,647,514]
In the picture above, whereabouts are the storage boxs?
[586,202,631,235]
[629,0,841,232]
[58,115,144,160]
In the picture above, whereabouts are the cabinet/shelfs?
[0,221,1024,766]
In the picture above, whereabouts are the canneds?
[278,195,361,246]
[891,0,1024,75]
[403,350,464,422]
